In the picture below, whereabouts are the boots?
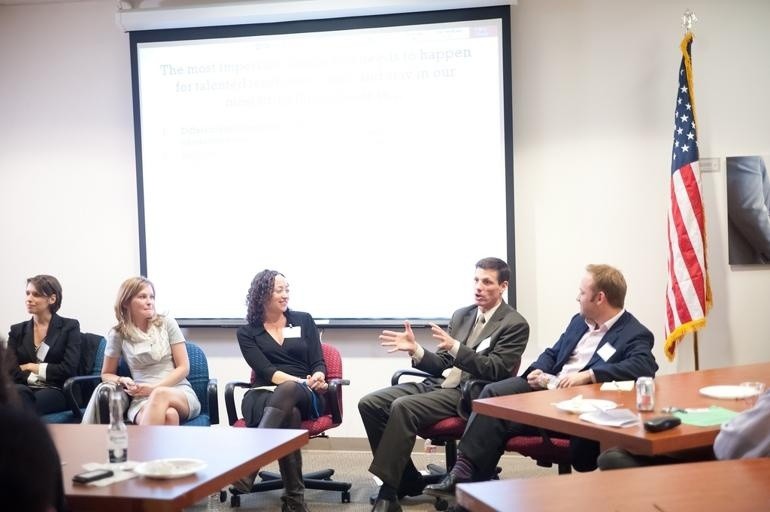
[277,448,310,512]
[229,405,288,494]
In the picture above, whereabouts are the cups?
[742,382,766,406]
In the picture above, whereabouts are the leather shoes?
[369,472,482,511]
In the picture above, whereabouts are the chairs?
[368,365,503,512]
[35,329,108,428]
[222,338,355,508]
[58,340,230,505]
[497,430,579,476]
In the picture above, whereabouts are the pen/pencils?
[612,380,624,392]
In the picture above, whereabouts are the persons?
[7,274,80,416]
[423,265,659,500]
[358,257,530,512]
[230,269,329,512]
[0,336,66,512]
[727,157,770,264]
[101,276,201,426]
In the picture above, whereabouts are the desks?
[447,454,770,511]
[470,358,770,461]
[43,418,312,512]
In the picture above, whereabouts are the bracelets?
[118,376,122,382]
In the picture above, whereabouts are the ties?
[440,313,485,389]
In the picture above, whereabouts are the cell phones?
[72,468,113,484]
[644,416,681,432]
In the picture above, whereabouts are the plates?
[133,458,204,480]
[700,384,757,400]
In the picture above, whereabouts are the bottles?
[537,372,561,390]
[105,392,129,467]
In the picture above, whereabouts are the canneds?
[635,377,655,411]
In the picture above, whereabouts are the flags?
[662,32,714,361]
